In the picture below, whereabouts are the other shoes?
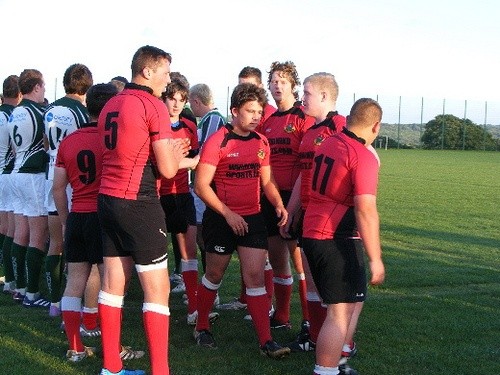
[186,309,220,326]
[258,338,290,360]
[250,315,291,330]
[24,296,51,307]
[100,366,146,375]
[192,327,217,349]
[171,281,186,293]
[283,334,316,353]
[214,298,249,311]
[341,341,356,359]
[299,319,309,335]
[119,344,145,360]
[12,290,25,300]
[169,272,182,282]
[47,305,60,317]
[78,324,102,338]
[243,304,274,320]
[66,346,97,361]
[3,283,14,293]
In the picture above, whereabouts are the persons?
[0,61,380,361]
[96,46,192,375]
[301,97,385,375]
[193,82,290,358]
